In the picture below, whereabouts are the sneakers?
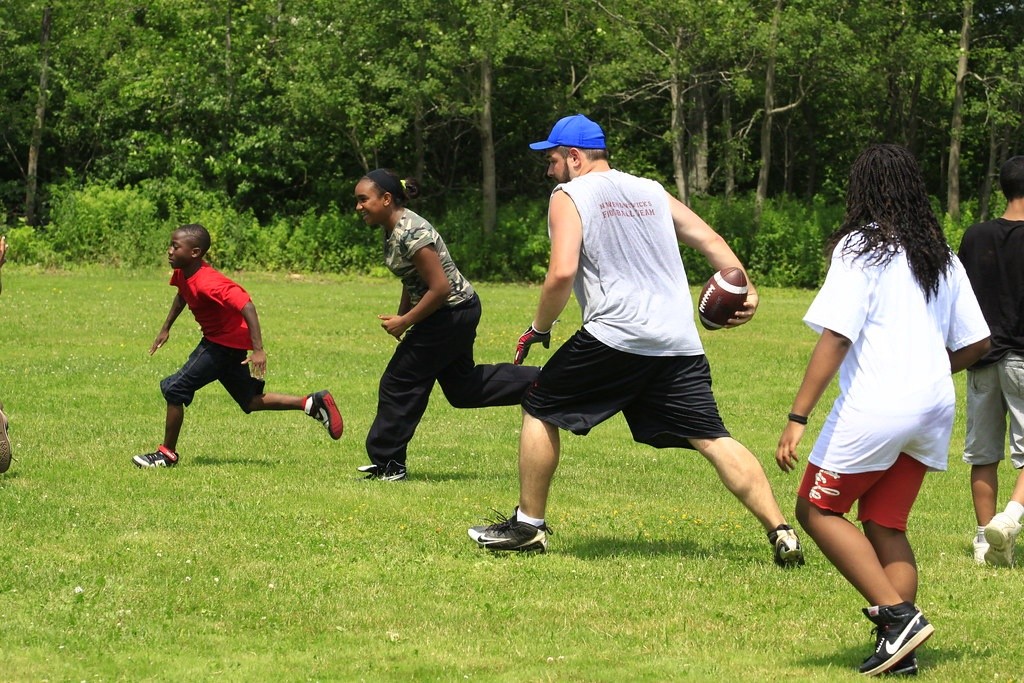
[356,458,408,484]
[131,445,181,469]
[973,535,991,566]
[0,410,13,474]
[766,523,804,568]
[854,598,935,677]
[984,511,1020,568]
[466,507,548,556]
[302,390,344,440]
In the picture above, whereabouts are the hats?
[530,115,606,153]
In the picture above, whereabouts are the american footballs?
[696,265,750,331]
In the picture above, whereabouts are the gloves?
[514,321,552,366]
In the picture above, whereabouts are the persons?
[467,115,807,571]
[130,225,344,468]
[775,143,992,676]
[956,155,1024,573]
[352,170,542,483]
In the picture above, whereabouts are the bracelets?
[788,413,808,425]
